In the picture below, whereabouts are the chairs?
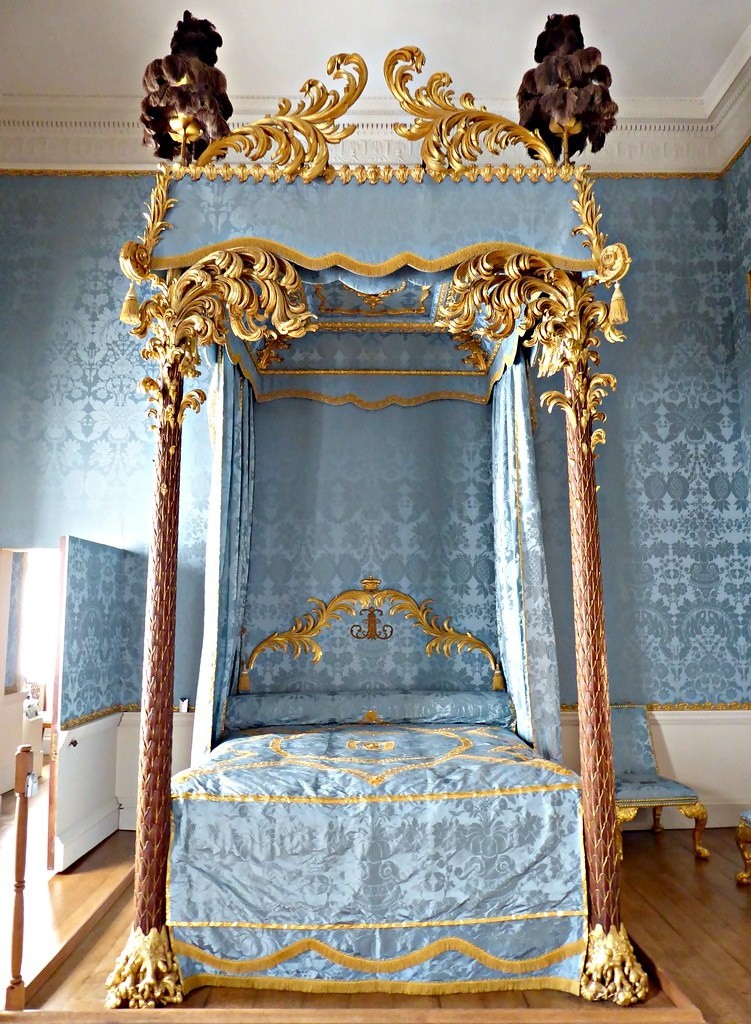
[611,705,710,861]
[731,811,751,890]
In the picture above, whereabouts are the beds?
[104,8,663,1010]
